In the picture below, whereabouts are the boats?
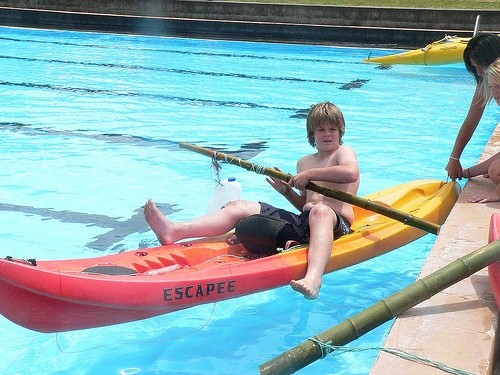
[362,34,476,66]
[0,180,461,334]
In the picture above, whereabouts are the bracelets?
[468,169,471,177]
[450,157,458,160]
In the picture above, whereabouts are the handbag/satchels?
[235,214,308,254]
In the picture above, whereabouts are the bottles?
[213,177,242,210]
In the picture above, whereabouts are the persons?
[446,35,500,181]
[464,59,500,203]
[143,102,360,298]
